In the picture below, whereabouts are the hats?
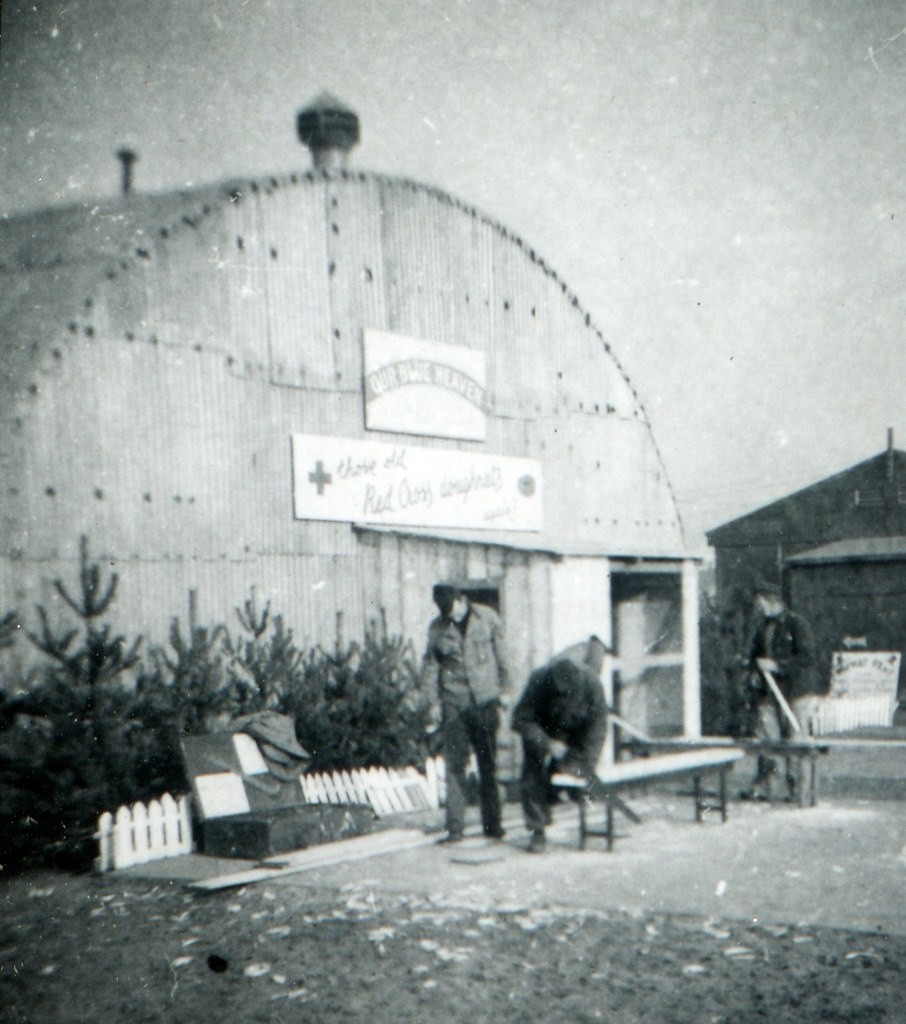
[433,584,457,616]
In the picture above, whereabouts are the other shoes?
[741,790,767,800]
[484,825,504,837]
[444,832,461,843]
[529,834,548,854]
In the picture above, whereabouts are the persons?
[735,582,816,805]
[508,658,608,853]
[420,584,515,846]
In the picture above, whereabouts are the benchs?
[552,747,745,850]
[617,737,834,808]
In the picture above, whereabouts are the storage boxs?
[179,733,372,860]
